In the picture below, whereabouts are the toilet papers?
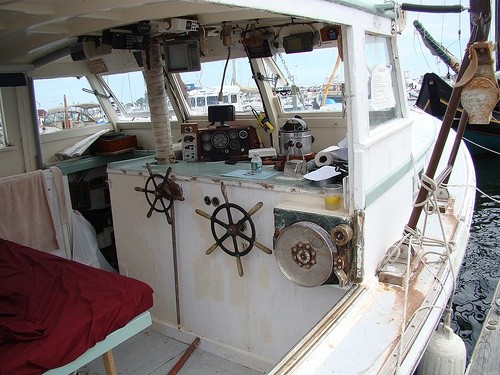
[315,146,339,168]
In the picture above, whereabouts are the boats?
[38,67,454,132]
[0,0,500,375]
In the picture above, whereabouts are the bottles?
[251,151,262,175]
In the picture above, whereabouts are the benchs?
[0,236,155,374]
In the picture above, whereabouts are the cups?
[323,184,342,210]
